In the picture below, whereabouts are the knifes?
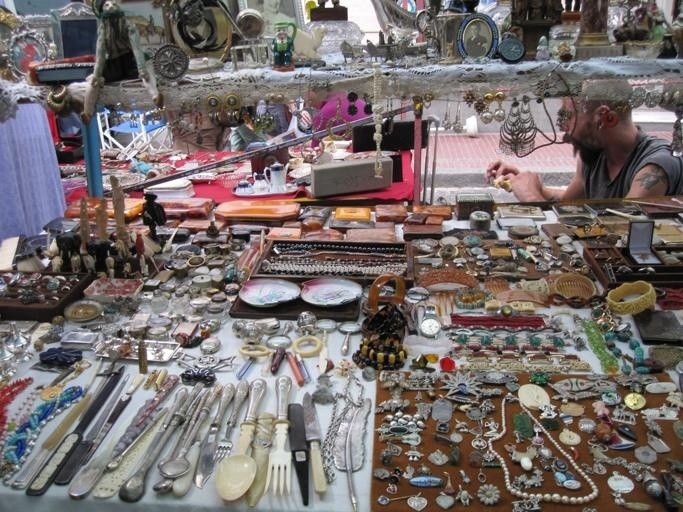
[302,392,328,495]
[194,381,236,490]
[287,402,310,506]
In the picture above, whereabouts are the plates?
[300,276,365,308]
[237,277,302,308]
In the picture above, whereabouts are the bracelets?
[352,335,409,370]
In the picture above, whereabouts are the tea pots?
[263,161,290,187]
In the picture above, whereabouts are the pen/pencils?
[271,347,311,386]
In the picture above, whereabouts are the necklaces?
[488,392,599,504]
[0,376,84,482]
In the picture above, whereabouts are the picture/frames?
[456,13,499,61]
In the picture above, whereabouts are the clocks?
[498,38,526,63]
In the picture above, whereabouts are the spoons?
[119,380,211,504]
[216,378,267,502]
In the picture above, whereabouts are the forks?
[262,376,292,497]
[211,380,249,465]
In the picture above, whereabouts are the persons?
[485,79,683,202]
[258,0,296,33]
[170,84,374,154]
[467,22,488,57]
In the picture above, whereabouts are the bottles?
[548,10,581,61]
[303,6,366,67]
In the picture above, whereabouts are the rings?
[0,271,79,305]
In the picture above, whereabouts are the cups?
[236,180,254,195]
[254,173,268,188]
